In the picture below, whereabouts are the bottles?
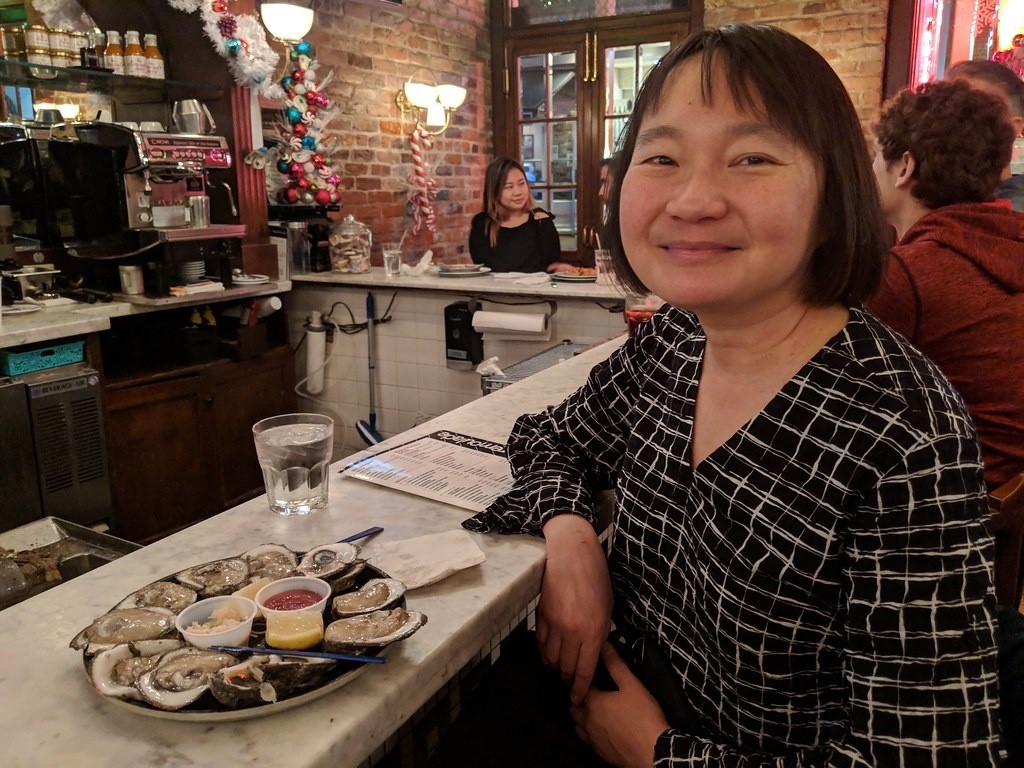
[0,25,165,80]
[330,214,372,274]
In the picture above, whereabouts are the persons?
[460,23,1008,768]
[469,158,561,274]
[869,79,1024,492]
[947,58,1024,213]
[547,158,613,273]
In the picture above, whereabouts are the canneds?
[0,24,91,78]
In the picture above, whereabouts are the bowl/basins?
[35,110,64,124]
[114,120,162,132]
[174,595,257,647]
[254,577,331,620]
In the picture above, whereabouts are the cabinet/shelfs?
[0,0,296,536]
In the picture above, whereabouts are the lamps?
[261,3,314,83]
[396,67,467,133]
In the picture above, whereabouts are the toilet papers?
[471,310,552,341]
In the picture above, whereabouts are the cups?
[189,196,210,228]
[625,297,659,337]
[381,242,403,277]
[252,412,334,516]
[119,265,144,294]
[171,99,206,135]
[594,249,618,285]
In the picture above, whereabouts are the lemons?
[266,611,324,650]
[231,577,275,618]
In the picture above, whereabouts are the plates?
[232,273,269,286]
[436,263,492,276]
[180,261,206,284]
[549,272,597,281]
[82,551,407,724]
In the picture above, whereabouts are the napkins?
[493,272,551,285]
[370,530,485,590]
[402,249,434,276]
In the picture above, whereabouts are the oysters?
[88,637,181,700]
[174,557,251,597]
[135,646,239,710]
[331,578,408,616]
[240,543,298,581]
[204,653,338,708]
[68,606,177,657]
[292,543,360,579]
[116,581,199,613]
[323,606,431,655]
